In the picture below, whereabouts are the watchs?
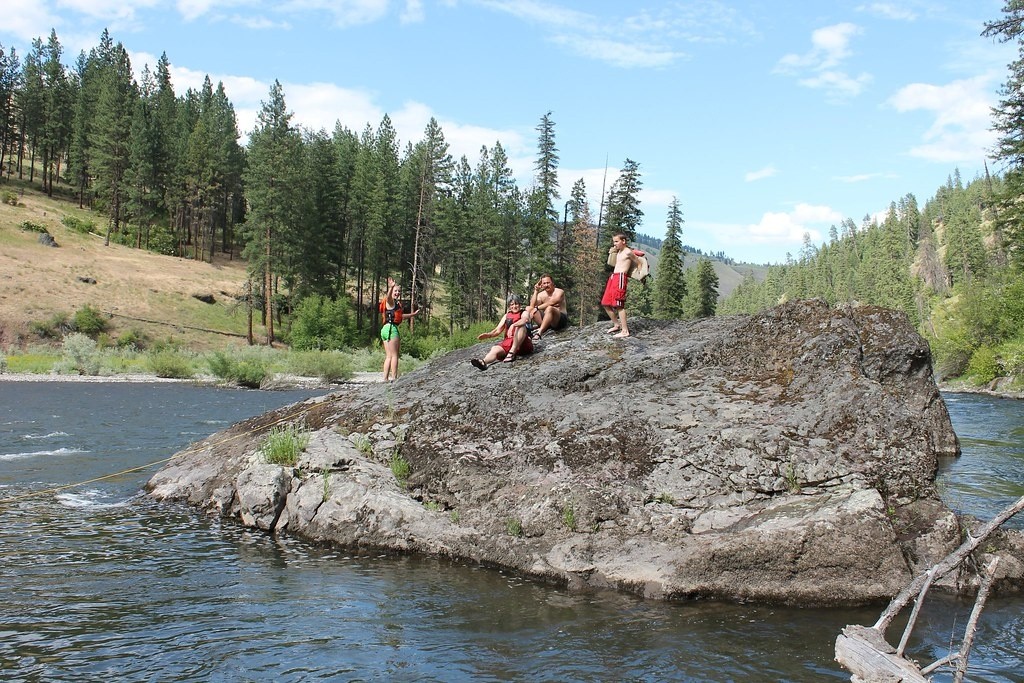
[535,306,539,310]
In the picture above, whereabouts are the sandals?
[471,357,487,370]
[504,352,516,363]
[532,334,541,341]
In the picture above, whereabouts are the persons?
[471,295,534,370]
[601,233,642,338]
[379,277,420,380]
[525,274,568,339]
